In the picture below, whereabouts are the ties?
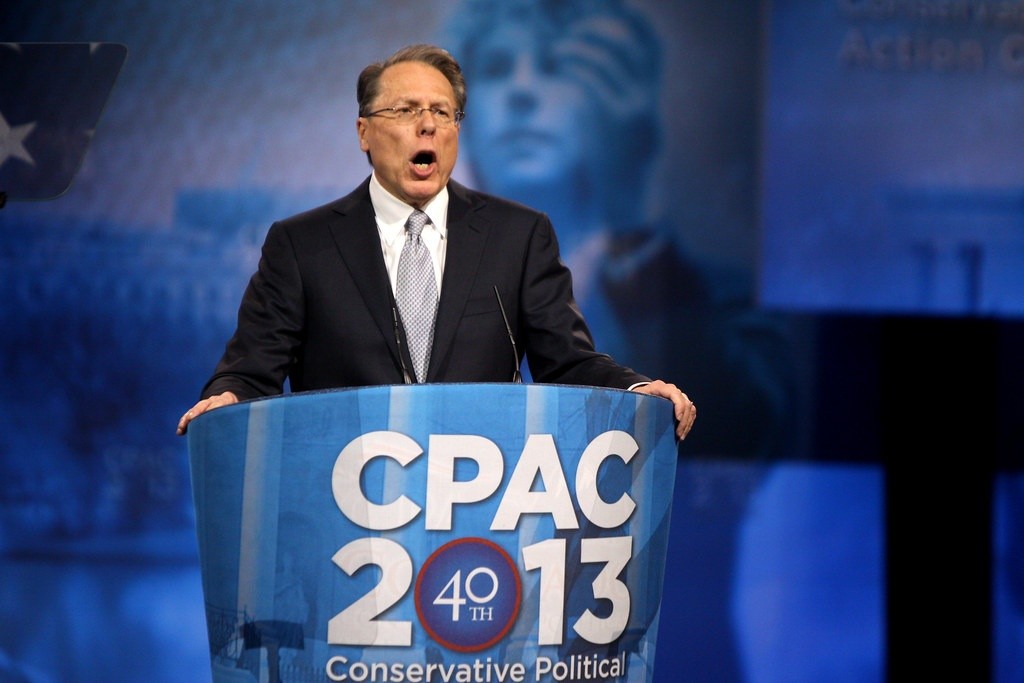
[395,210,437,383]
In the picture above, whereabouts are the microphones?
[387,284,413,384]
[494,285,523,384]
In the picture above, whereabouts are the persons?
[430,0,798,464]
[176,44,697,441]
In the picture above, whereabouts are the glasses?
[366,105,464,127]
